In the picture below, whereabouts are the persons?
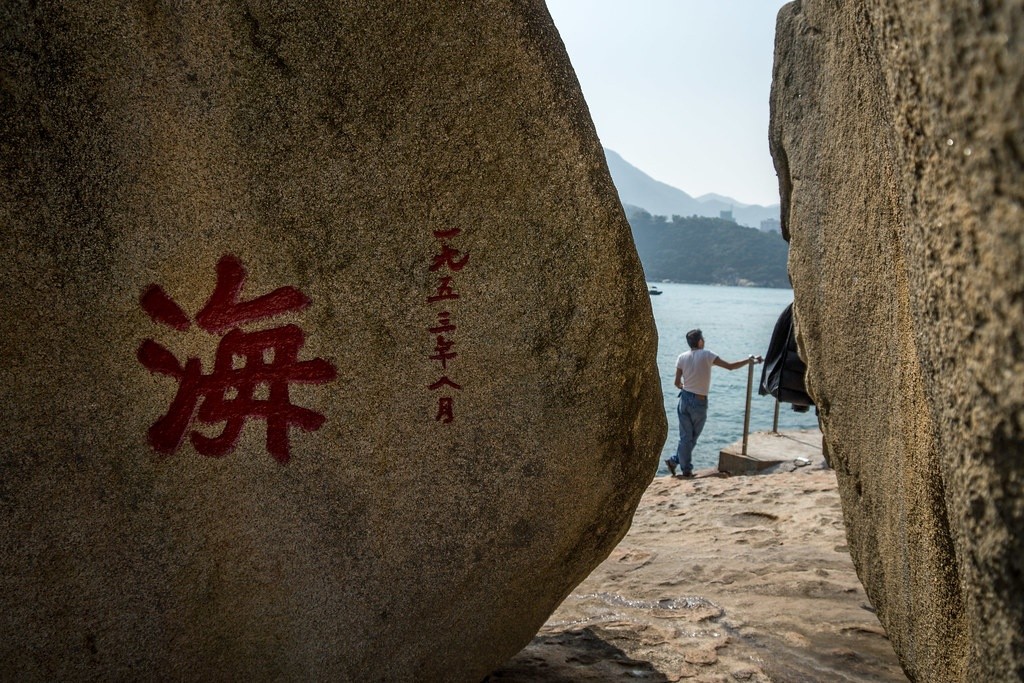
[664,328,755,477]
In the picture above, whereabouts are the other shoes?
[683,470,694,478]
[665,458,677,477]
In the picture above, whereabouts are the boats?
[648,286,664,296]
